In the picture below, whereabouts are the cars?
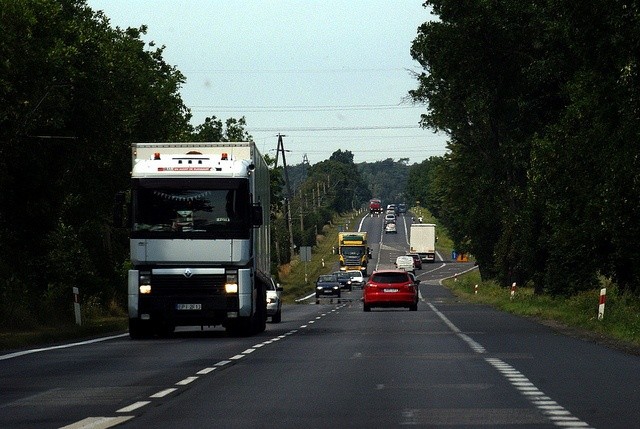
[387,221,394,224]
[266,277,283,323]
[345,270,364,289]
[386,224,397,234]
[313,275,341,298]
[387,203,407,216]
[332,271,352,292]
[367,247,373,259]
[408,273,418,292]
[363,270,418,311]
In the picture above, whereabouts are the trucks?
[127,142,271,336]
[370,198,381,214]
[338,232,368,278]
[410,224,437,263]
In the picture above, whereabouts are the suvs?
[406,254,422,270]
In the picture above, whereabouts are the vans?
[385,215,395,224]
[394,256,415,276]
